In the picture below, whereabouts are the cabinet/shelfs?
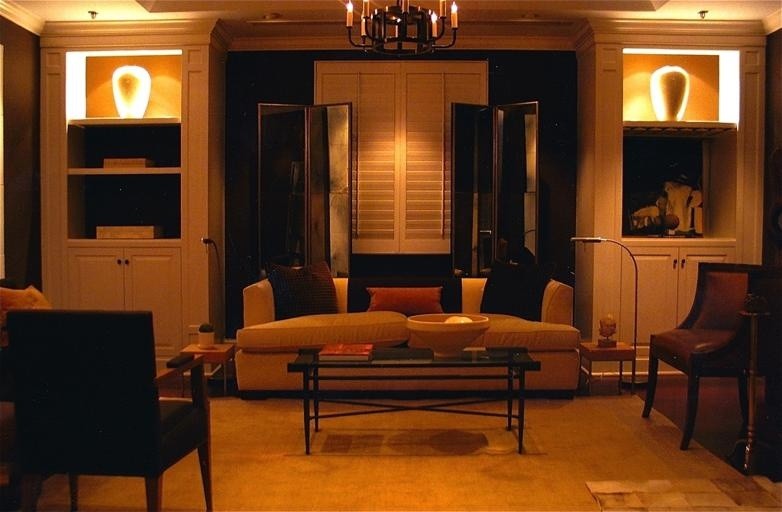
[578,48,762,360]
[41,36,224,376]
[314,61,490,255]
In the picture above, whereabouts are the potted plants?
[597,314,618,347]
[198,323,219,349]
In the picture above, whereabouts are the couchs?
[235,278,578,390]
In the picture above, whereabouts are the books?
[317,343,373,361]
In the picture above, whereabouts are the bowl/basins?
[406,313,490,359]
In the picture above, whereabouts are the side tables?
[579,341,636,394]
[180,341,236,396]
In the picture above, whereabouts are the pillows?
[367,285,445,314]
[479,266,550,322]
[266,260,339,320]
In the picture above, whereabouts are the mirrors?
[451,101,538,278]
[259,100,350,280]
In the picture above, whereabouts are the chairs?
[1,307,215,510]
[640,260,779,450]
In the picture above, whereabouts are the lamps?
[569,234,638,395]
[343,0,460,61]
[200,237,232,388]
